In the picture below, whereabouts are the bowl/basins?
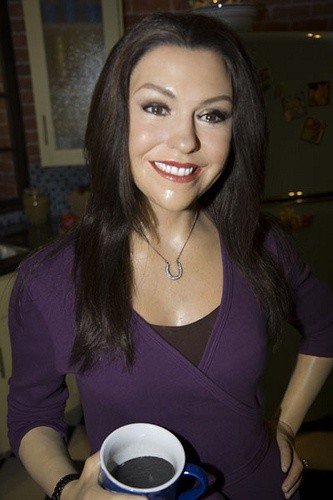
[191,4,255,34]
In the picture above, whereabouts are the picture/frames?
[23,0,124,169]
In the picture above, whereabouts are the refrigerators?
[239,31,332,426]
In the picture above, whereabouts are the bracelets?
[50,473,80,500]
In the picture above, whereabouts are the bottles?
[29,219,55,249]
[23,188,50,224]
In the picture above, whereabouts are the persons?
[6,9,333,500]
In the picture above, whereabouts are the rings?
[301,455,312,468]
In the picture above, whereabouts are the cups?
[99,422,208,500]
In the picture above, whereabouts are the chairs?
[0,239,84,458]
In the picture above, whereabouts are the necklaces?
[137,209,200,280]
